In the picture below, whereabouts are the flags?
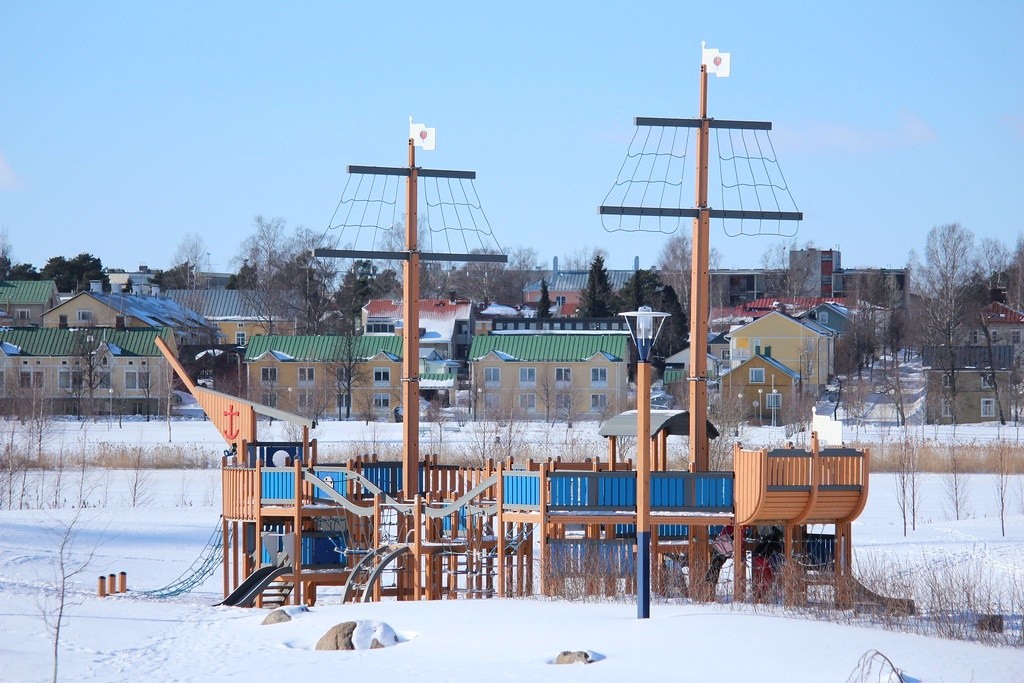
[411,125,436,149]
[703,47,730,75]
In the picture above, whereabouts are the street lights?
[757,388,763,425]
[618,306,674,619]
[738,393,743,422]
[109,389,114,428]
[478,388,482,424]
[288,386,293,413]
